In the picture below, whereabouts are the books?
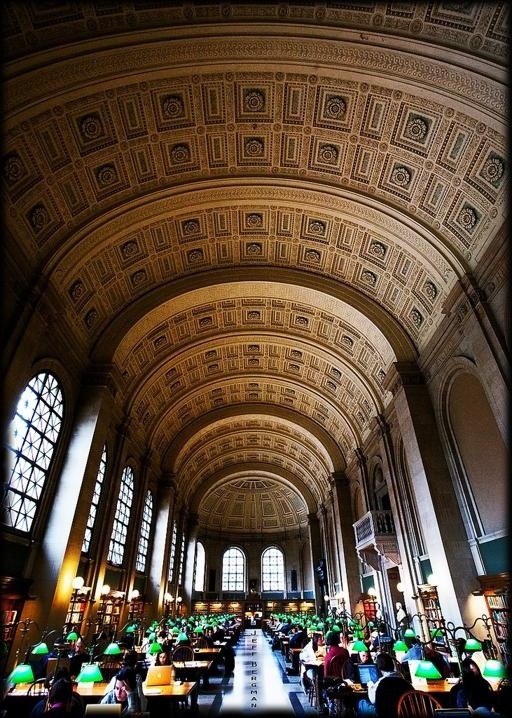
[424,599,448,649]
[128,601,144,621]
[95,603,122,643]
[484,594,511,660]
[364,603,376,622]
[64,594,86,633]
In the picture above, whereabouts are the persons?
[327,604,333,617]
[4,610,238,716]
[374,602,385,636]
[395,600,406,642]
[251,609,489,716]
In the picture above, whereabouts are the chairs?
[282,622,511,717]
[1,624,234,717]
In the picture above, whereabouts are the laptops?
[356,663,379,688]
[84,703,122,718]
[145,665,173,686]
